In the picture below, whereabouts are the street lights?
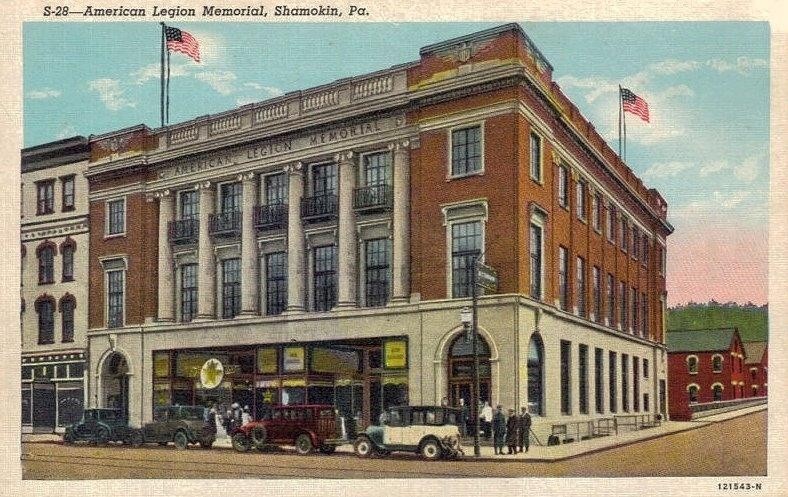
[460,305,483,460]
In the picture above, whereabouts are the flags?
[621,88,651,124]
[163,26,201,63]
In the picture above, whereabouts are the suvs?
[229,403,343,455]
[129,404,216,449]
[354,405,465,461]
[63,407,133,447]
